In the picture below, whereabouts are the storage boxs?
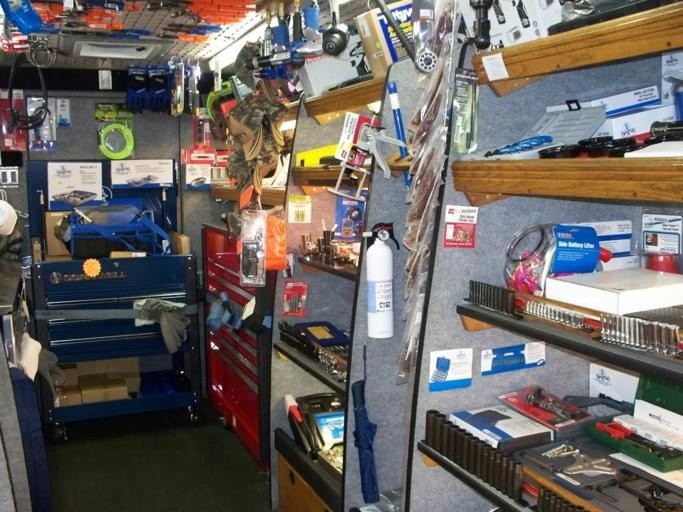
[353,0,414,79]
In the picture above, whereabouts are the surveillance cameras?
[321,28,348,56]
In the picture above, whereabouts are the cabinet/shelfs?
[211,57,449,511]
[33,234,207,442]
[400,0,683,512]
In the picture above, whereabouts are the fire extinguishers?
[362,221,399,340]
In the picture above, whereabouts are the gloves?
[127,71,147,114]
[149,71,171,112]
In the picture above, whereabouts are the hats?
[225,76,286,210]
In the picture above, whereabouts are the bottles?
[503,224,604,297]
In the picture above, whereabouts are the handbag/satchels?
[70,204,175,258]
